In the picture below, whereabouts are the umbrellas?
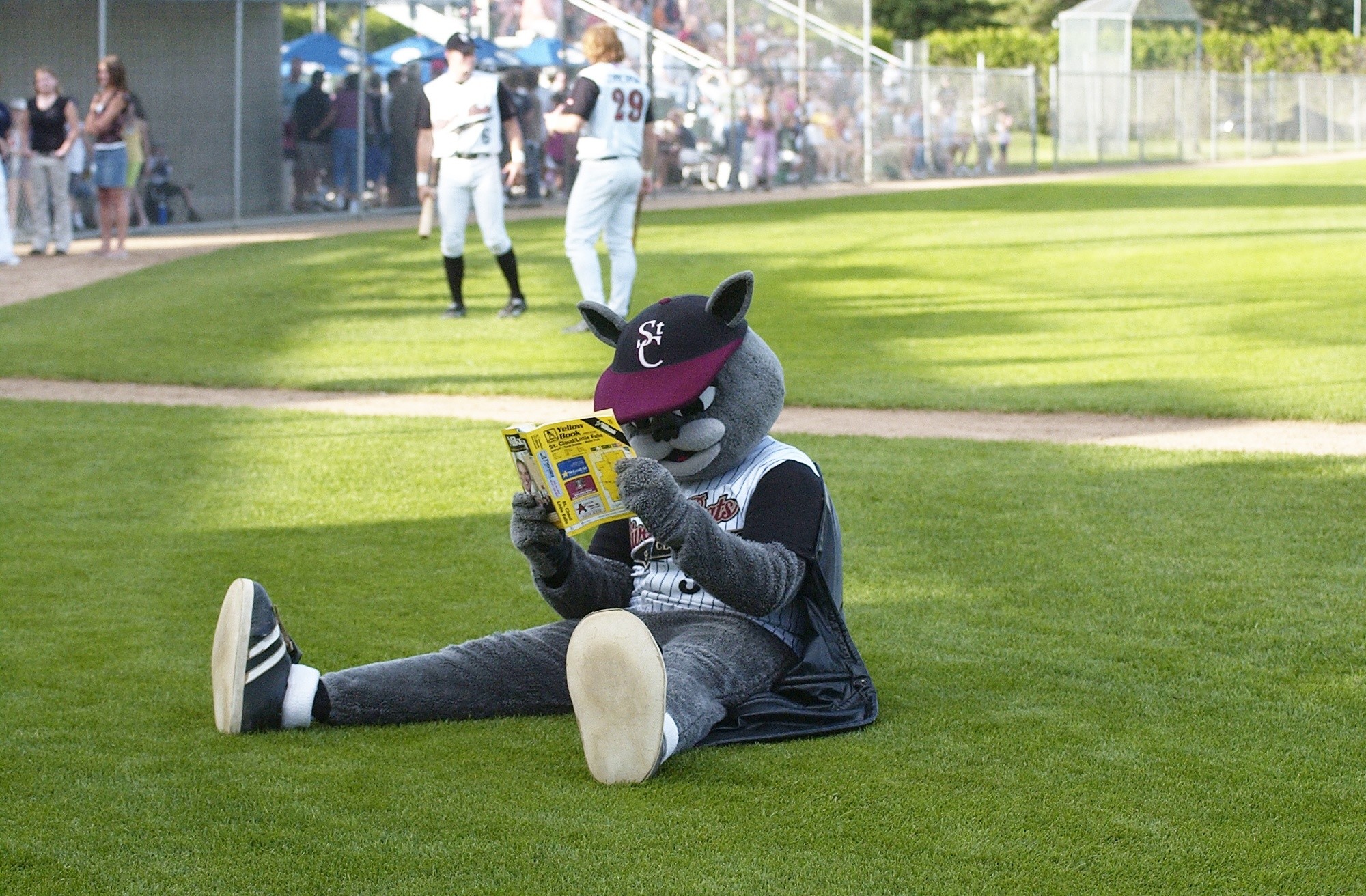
[370,34,446,65]
[425,38,525,69]
[518,31,590,67]
[279,31,387,74]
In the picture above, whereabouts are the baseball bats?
[417,158,437,239]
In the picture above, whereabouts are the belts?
[599,156,616,160]
[455,153,478,159]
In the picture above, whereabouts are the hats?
[445,32,478,52]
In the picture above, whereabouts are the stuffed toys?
[210,266,882,789]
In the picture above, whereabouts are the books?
[499,408,638,539]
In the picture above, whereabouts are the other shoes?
[500,297,524,318]
[444,304,463,318]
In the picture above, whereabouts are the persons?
[414,30,528,321]
[82,54,134,257]
[284,0,1018,212]
[561,20,658,334]
[0,54,151,268]
[18,64,79,258]
